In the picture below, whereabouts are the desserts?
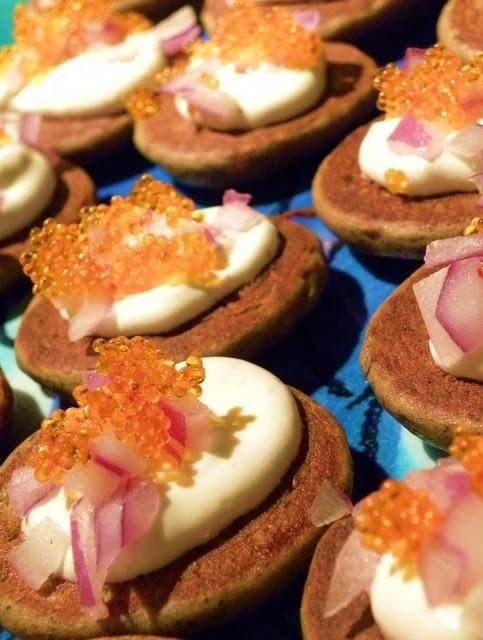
[0,0,483,640]
